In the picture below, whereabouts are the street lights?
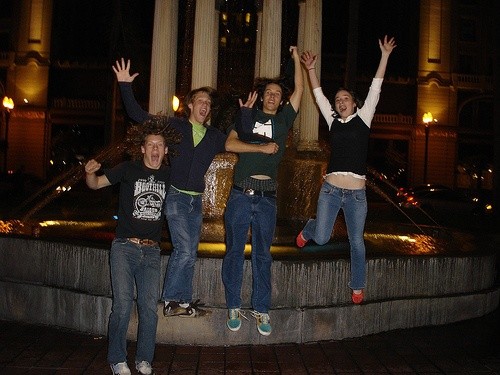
[0,95,15,216]
[422,111,433,184]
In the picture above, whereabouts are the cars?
[391,183,500,230]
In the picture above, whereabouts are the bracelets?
[308,67,316,70]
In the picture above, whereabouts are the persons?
[49,129,76,220]
[112,57,257,319]
[221,45,304,336]
[299,36,397,304]
[84,128,174,375]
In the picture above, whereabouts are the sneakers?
[227,308,249,331]
[136,360,152,375]
[110,361,131,375]
[249,309,272,336]
[179,298,212,318]
[163,301,188,317]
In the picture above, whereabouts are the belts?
[233,184,276,196]
[127,237,159,246]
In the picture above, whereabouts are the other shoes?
[352,289,363,304]
[296,231,308,247]
